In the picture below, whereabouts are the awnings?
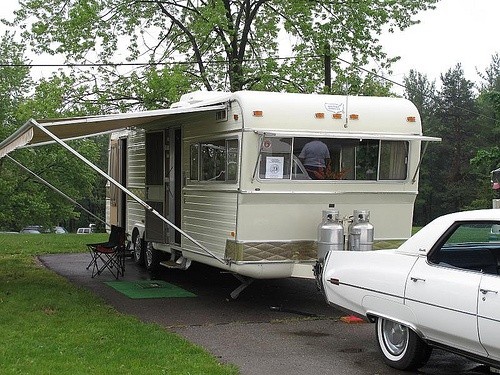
[0,105,230,264]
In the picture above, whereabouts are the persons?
[299,138,330,180]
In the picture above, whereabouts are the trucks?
[0,90,441,300]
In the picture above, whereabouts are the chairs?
[85,224,131,281]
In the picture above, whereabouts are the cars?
[20,224,97,235]
[321,208,500,372]
